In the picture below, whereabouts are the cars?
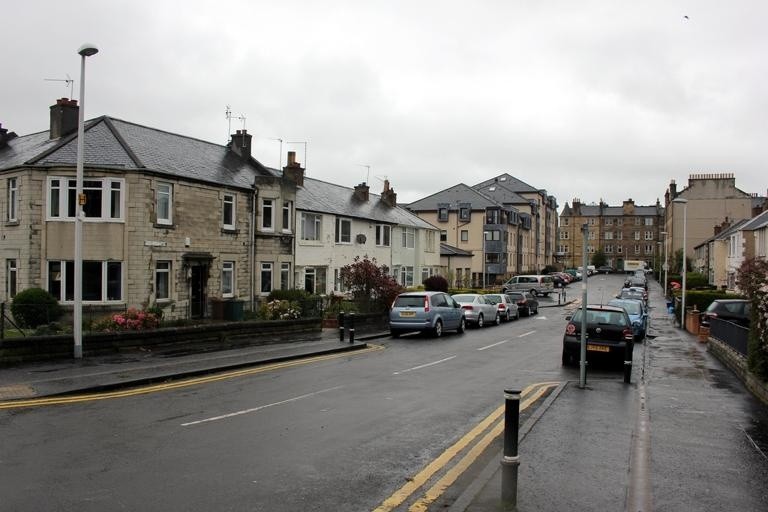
[451,292,502,328]
[596,267,617,274]
[608,269,649,340]
[388,290,466,338]
[505,291,540,317]
[699,297,754,328]
[482,293,521,322]
[546,265,598,289]
[559,303,642,375]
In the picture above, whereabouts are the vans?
[501,274,555,298]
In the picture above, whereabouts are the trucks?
[624,260,653,275]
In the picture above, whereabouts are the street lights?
[671,197,689,330]
[660,230,671,298]
[73,43,99,358]
[656,241,663,284]
[483,230,489,289]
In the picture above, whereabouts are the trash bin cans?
[212,297,243,321]
